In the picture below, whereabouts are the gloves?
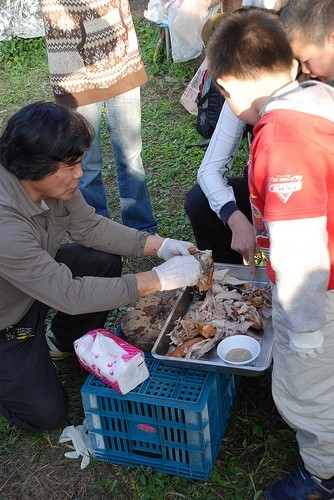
[287,330,324,360]
[157,237,200,262]
[152,255,202,291]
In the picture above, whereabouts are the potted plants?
[79,330,238,481]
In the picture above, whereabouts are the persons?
[33,0,161,233]
[206,10,334,500]
[184,0,334,280]
[0,101,204,436]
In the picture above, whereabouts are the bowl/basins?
[215,334,261,366]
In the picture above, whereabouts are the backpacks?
[195,69,249,141]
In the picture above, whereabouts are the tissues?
[73,328,152,395]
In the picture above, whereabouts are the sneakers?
[264,460,334,500]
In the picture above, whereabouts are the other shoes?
[45,324,76,360]
